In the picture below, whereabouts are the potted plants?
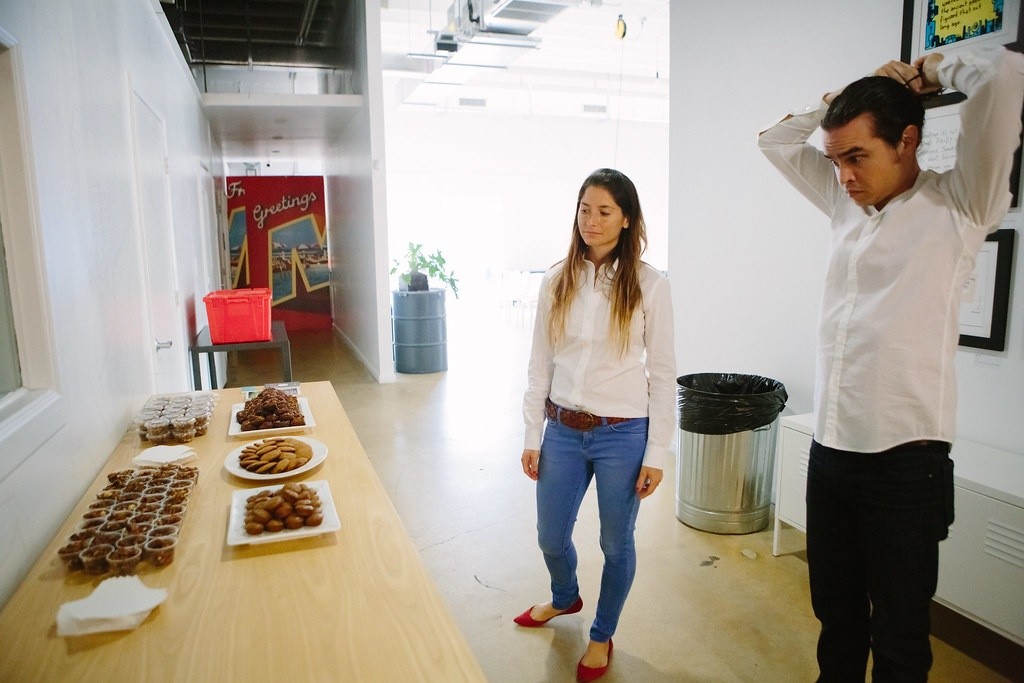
[390,240,460,301]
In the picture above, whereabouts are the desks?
[191,320,292,390]
[0,381,487,683]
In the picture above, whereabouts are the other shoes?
[575,638,613,683]
[513,596,583,628]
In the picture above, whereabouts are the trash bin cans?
[674,372,786,535]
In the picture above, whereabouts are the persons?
[755,52,1023,683]
[514,169,677,683]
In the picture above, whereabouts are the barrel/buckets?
[393,287,449,374]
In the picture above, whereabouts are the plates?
[228,397,315,436]
[223,436,329,480]
[224,479,342,545]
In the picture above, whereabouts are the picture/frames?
[900,0,1024,67]
[921,91,1024,208]
[958,228,1016,351]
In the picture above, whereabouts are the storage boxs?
[204,287,273,344]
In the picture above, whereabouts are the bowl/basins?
[57,465,199,574]
[134,388,216,445]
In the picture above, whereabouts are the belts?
[545,397,636,432]
[901,440,938,447]
